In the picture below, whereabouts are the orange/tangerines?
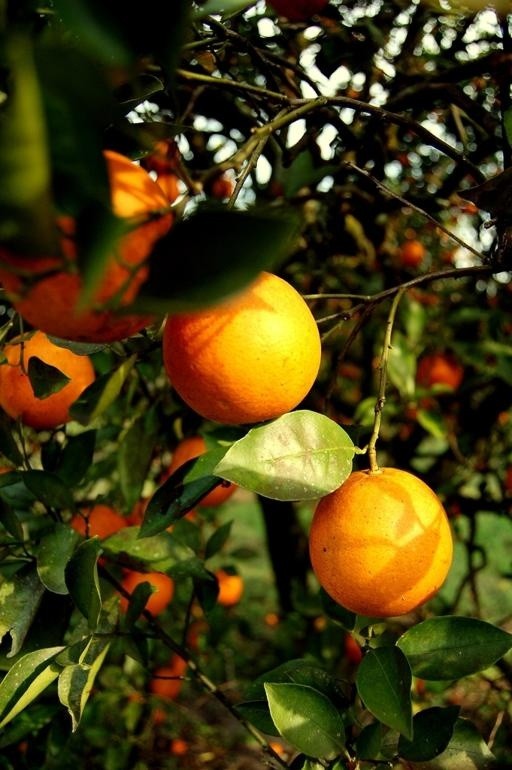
[0,0,511,759]
